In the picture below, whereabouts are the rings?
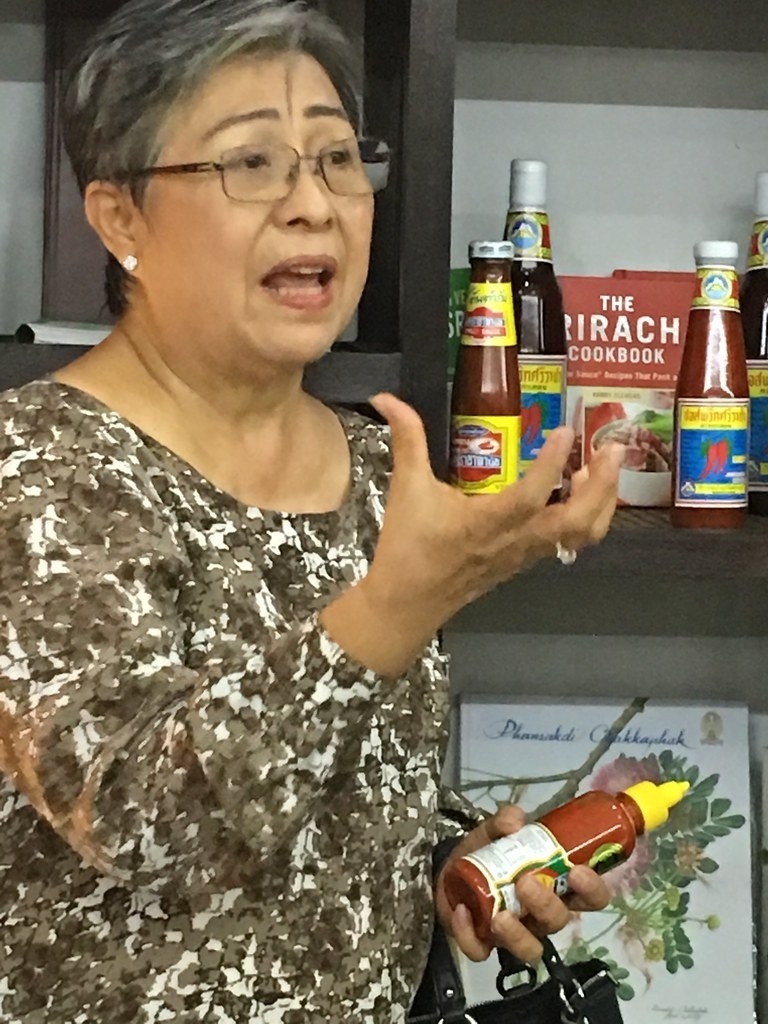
[555,540,577,567]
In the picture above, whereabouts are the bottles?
[502,159,567,505]
[442,781,689,938]
[737,172,768,515]
[448,241,519,495]
[669,239,751,529]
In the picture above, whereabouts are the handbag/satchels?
[405,806,624,1024]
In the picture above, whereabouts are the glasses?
[105,139,390,204]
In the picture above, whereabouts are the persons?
[0,0,628,1024]
